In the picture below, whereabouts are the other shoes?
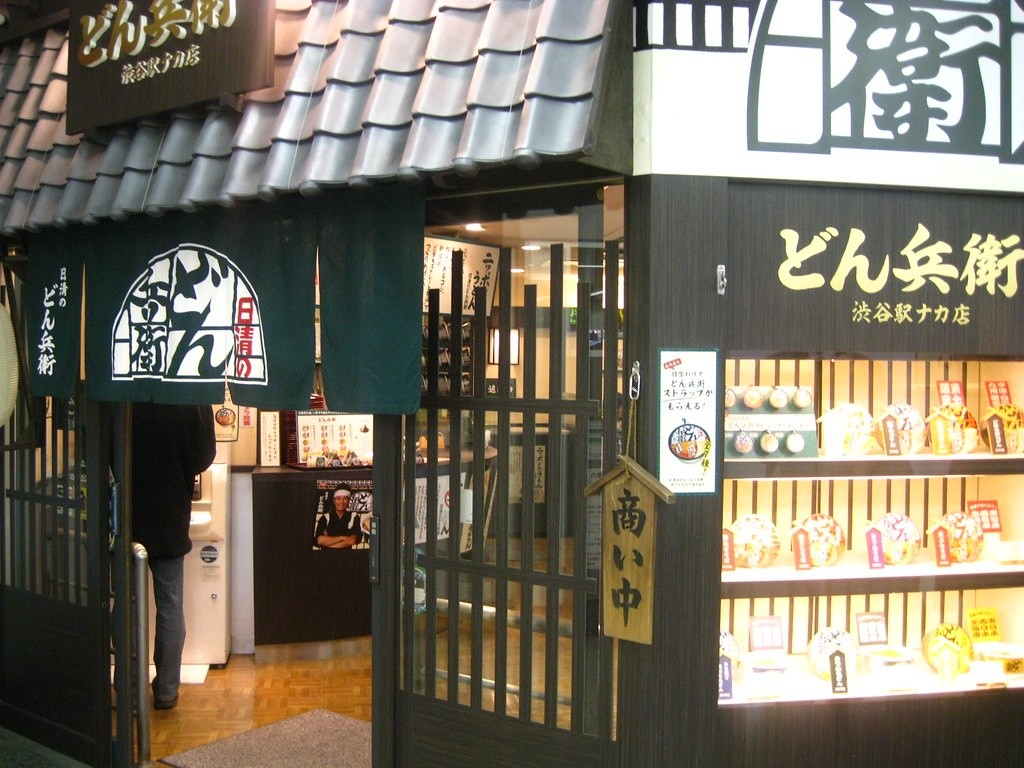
[155,692,178,708]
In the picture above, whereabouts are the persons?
[82,401,218,715]
[314,482,363,551]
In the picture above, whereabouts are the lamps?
[488,328,521,365]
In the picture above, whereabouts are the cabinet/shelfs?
[717,452,1024,713]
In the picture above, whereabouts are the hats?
[333,483,351,498]
